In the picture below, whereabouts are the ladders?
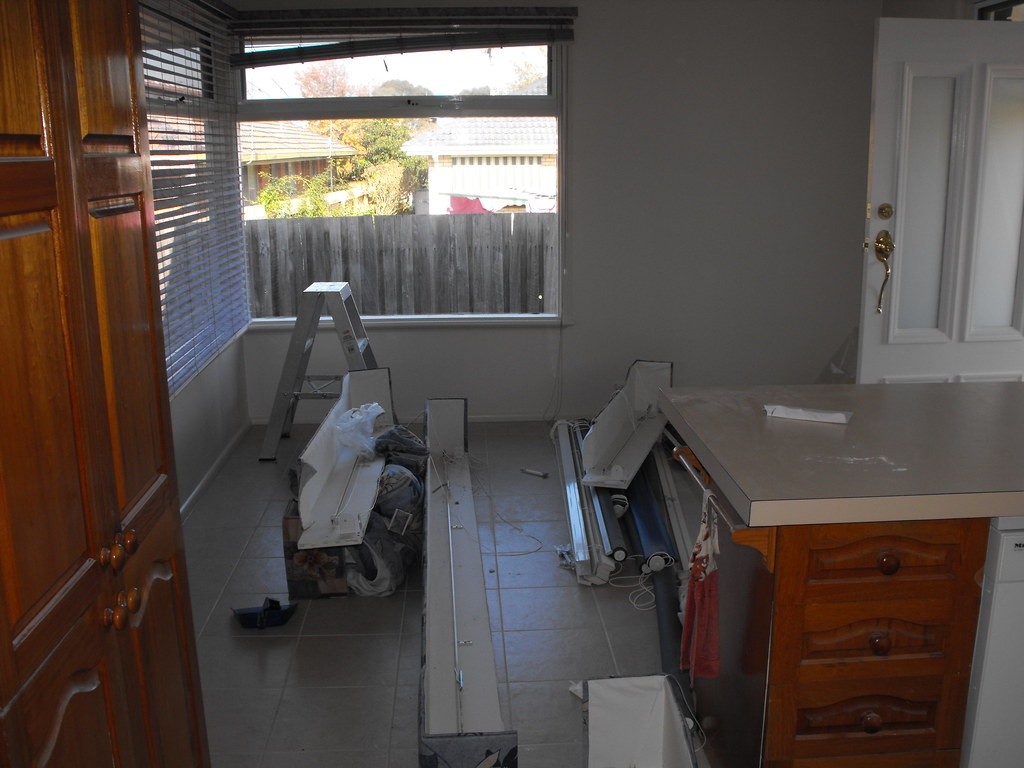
[257,282,399,462]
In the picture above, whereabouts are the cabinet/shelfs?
[0,0,212,768]
[662,424,991,768]
